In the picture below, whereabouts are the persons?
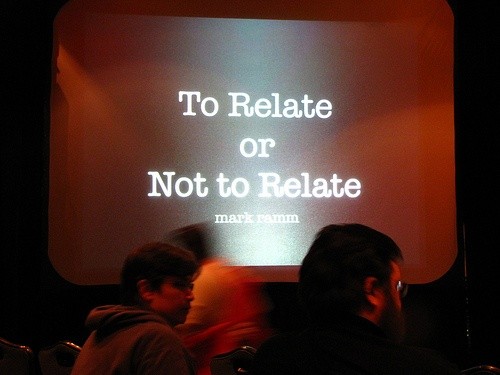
[170,225,271,374]
[252,224,445,375]
[70,242,196,375]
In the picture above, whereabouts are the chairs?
[39,340,81,375]
[0,338,34,375]
[210,345,258,375]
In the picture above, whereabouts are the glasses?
[158,279,194,292]
[388,276,408,299]
